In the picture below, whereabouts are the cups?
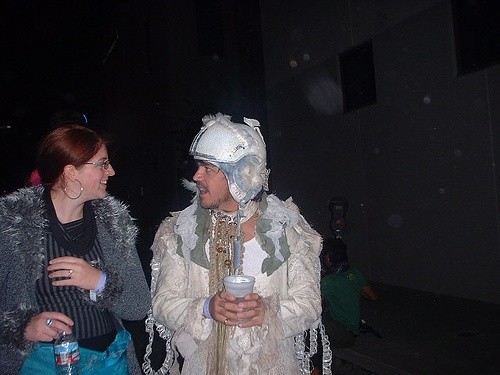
[223,276,256,324]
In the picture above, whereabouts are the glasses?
[82,159,109,171]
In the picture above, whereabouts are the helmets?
[189,116,269,203]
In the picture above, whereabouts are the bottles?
[53,325,81,375]
